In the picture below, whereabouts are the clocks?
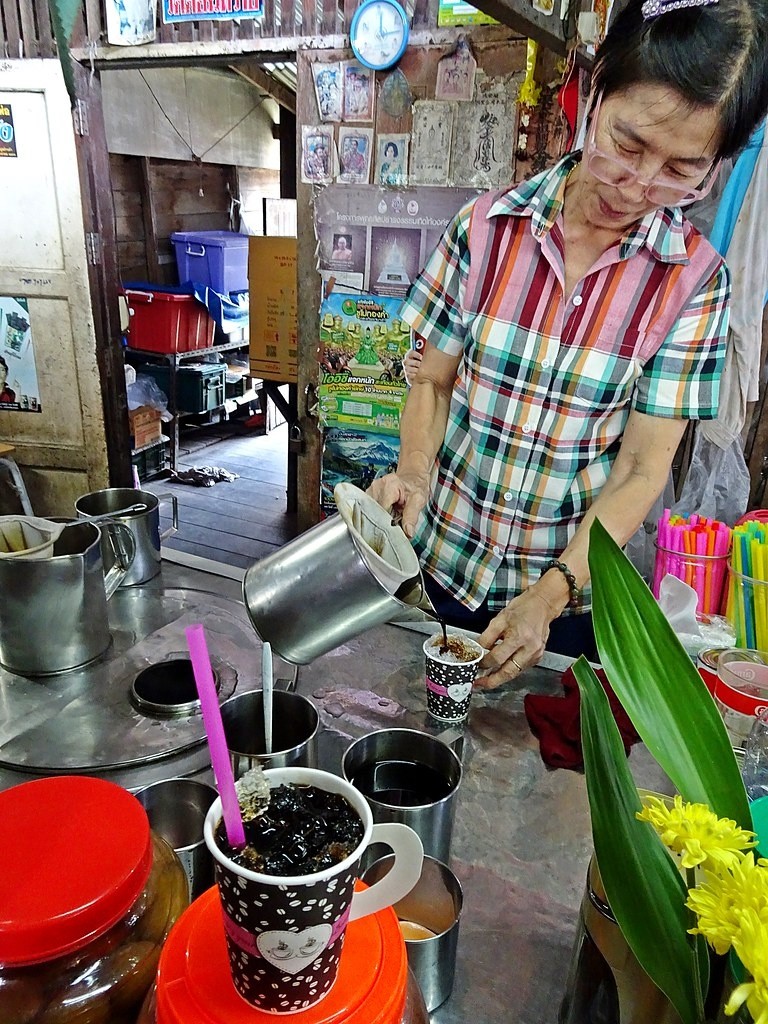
[349,0,409,70]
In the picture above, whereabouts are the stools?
[0,442,34,517]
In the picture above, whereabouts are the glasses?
[586,84,722,208]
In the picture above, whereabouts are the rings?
[512,659,523,672]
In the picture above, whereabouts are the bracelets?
[539,560,579,610]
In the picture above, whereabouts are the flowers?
[569,513,768,1024]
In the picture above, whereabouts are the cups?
[699,645,768,803]
[0,515,136,677]
[203,765,424,1014]
[74,487,179,588]
[131,778,220,906]
[359,854,464,1014]
[218,678,320,782]
[558,852,681,1024]
[423,633,492,724]
[339,726,464,867]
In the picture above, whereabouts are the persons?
[365,0,768,691]
[0,355,21,402]
[331,237,352,260]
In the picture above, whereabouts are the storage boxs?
[129,406,162,448]
[132,438,165,482]
[247,236,298,384]
[169,229,249,293]
[139,361,227,415]
[123,283,216,354]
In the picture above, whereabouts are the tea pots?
[242,506,442,667]
